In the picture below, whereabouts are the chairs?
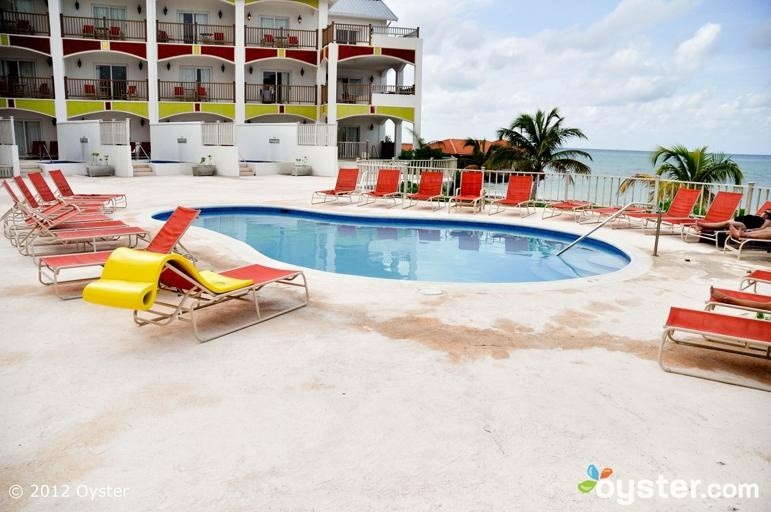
[312,164,771,397]
[0,169,311,345]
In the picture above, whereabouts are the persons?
[260,85,273,104]
[695,209,771,232]
[729,224,771,238]
